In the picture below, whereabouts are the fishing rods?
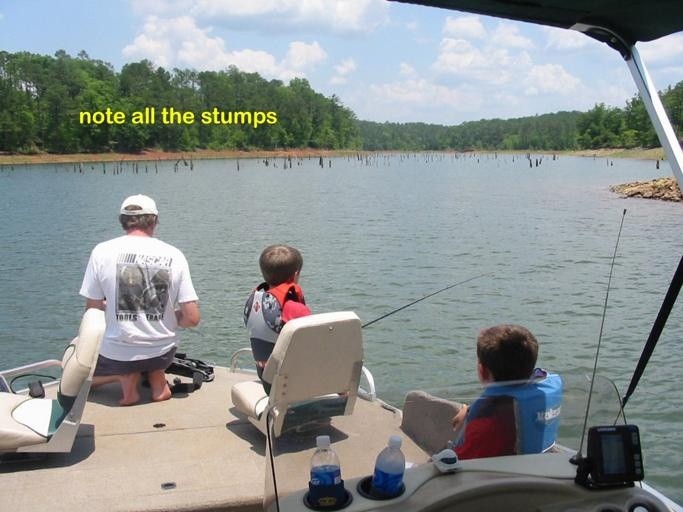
[362,271,495,329]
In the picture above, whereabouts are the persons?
[79,190,201,407]
[241,242,317,401]
[441,323,564,459]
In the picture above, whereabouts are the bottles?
[308,434,348,509]
[370,434,407,498]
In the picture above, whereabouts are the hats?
[118,193,157,217]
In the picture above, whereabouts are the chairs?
[0,308,106,453]
[231,311,365,437]
